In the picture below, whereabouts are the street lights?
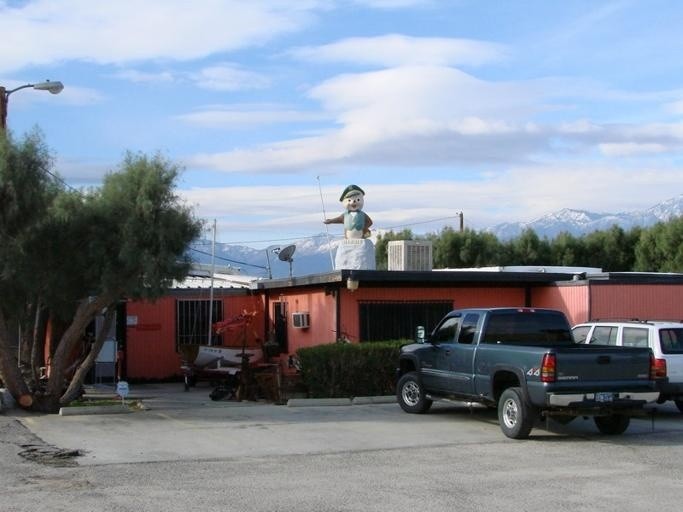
[0,80,64,128]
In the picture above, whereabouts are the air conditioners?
[386,239,433,273]
[292,311,308,330]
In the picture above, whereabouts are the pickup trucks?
[396,307,669,439]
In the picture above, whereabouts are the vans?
[570,317,683,413]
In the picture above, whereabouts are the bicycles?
[180,355,194,391]
